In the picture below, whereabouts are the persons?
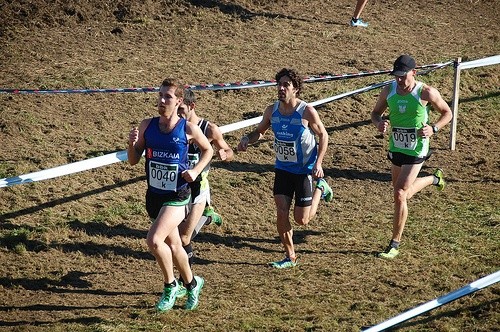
[175,88,234,298]
[351,0,369,26]
[371,55,452,258]
[238,67,334,269]
[127,78,214,313]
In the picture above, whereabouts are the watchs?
[431,124,438,133]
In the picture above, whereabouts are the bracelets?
[240,136,249,143]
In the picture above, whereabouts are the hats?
[388,55,415,76]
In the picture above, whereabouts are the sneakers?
[158,279,179,311]
[435,168,445,192]
[203,205,223,227]
[351,19,367,27]
[186,276,204,309]
[379,247,399,259]
[176,280,188,297]
[272,257,297,270]
[316,178,333,203]
